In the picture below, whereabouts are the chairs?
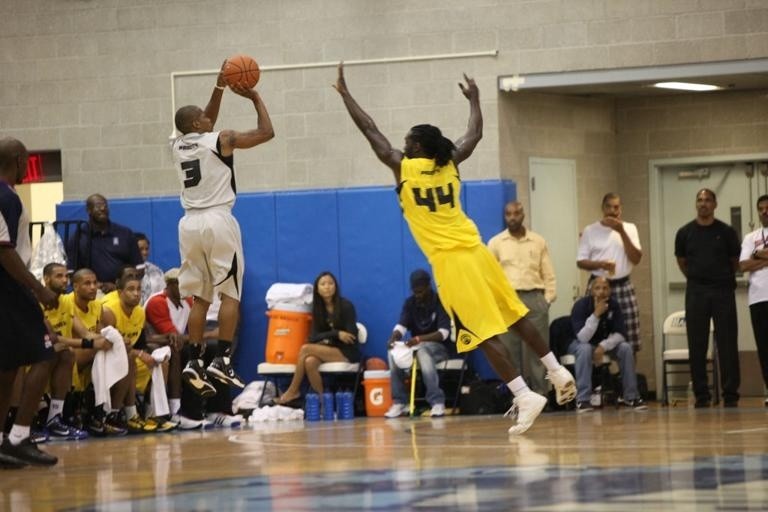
[549,316,620,411]
[256,362,310,411]
[316,321,368,403]
[661,310,720,407]
[415,352,474,415]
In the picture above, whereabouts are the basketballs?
[364,358,387,371]
[222,55,261,90]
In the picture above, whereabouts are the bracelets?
[213,83,227,91]
[80,337,95,350]
[413,333,421,344]
[752,249,760,260]
[137,348,144,361]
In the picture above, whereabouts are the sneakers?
[503,366,648,434]
[431,404,445,416]
[384,403,416,417]
[207,356,245,390]
[0,413,243,467]
[182,359,217,398]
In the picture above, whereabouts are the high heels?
[272,393,305,405]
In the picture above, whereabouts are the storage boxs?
[361,369,394,417]
[264,310,314,364]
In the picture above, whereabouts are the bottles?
[323,386,334,420]
[342,388,353,418]
[335,387,343,418]
[310,390,319,421]
[305,387,313,421]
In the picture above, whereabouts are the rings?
[605,303,609,307]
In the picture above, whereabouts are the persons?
[167,53,278,398]
[331,58,579,441]
[560,276,644,409]
[483,199,558,411]
[385,269,454,420]
[737,195,768,409]
[66,194,145,294]
[272,272,361,420]
[577,191,643,408]
[34,230,255,445]
[673,187,742,410]
[1,130,57,475]
